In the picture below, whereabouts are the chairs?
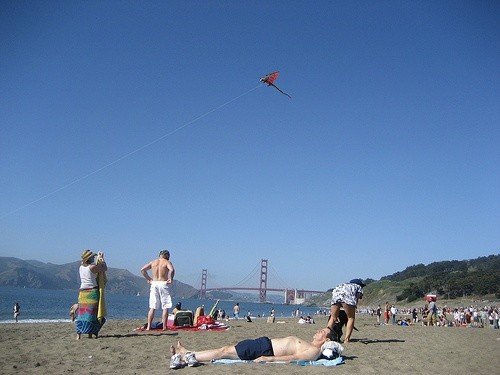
[174,311,194,327]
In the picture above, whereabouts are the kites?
[259,71,292,98]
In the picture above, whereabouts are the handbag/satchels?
[193,305,204,327]
[174,310,194,326]
[143,322,163,330]
[197,315,214,326]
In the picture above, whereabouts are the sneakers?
[170,354,185,369]
[182,352,200,367]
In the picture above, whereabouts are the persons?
[141,250,175,331]
[327,279,364,344]
[170,327,337,362]
[75,249,107,339]
[13,297,500,328]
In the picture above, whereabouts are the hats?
[80,250,97,264]
[350,279,366,288]
